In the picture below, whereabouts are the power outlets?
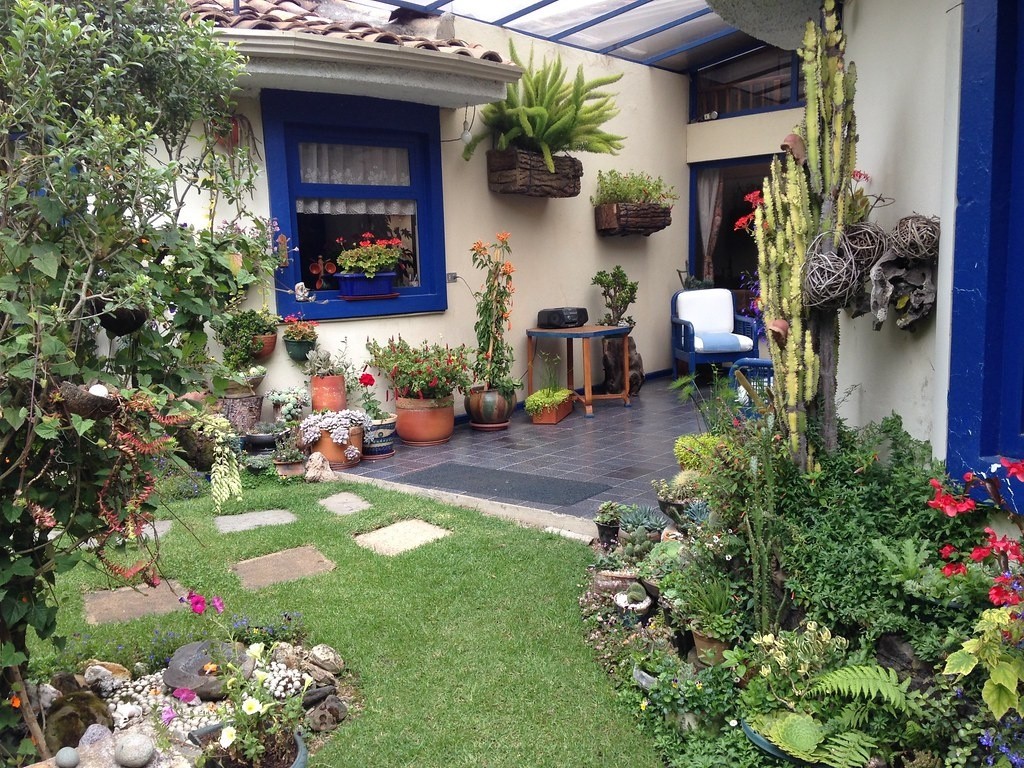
[446,272,457,282]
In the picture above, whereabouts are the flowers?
[354,374,391,420]
[589,169,680,210]
[734,0,871,475]
[739,269,760,298]
[179,213,299,272]
[927,457,1024,651]
[467,231,524,406]
[336,232,402,278]
[150,590,314,768]
[365,333,477,402]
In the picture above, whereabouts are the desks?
[526,326,634,417]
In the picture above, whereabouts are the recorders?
[538,306,589,329]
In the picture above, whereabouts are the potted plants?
[209,282,283,395]
[301,336,364,414]
[210,107,263,219]
[461,38,627,199]
[523,350,574,425]
[179,413,248,514]
[246,386,375,478]
[593,469,927,768]
[94,273,155,335]
[590,266,639,337]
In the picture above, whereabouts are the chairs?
[670,287,760,391]
[727,358,775,420]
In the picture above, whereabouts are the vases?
[594,203,672,237]
[363,412,398,455]
[396,397,454,442]
[213,272,258,307]
[333,272,397,295]
[218,720,308,768]
[464,385,518,423]
[748,297,759,318]
